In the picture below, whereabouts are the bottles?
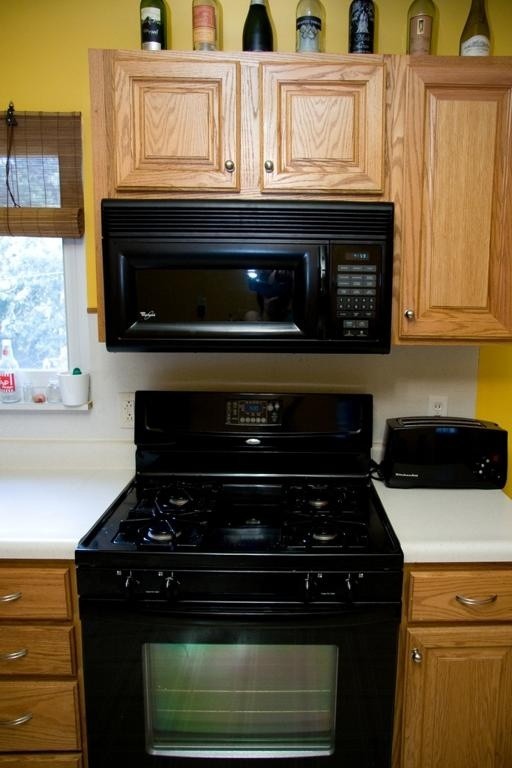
[294,0,324,53]
[138,0,167,51]
[460,0,492,57]
[406,0,439,53]
[242,0,276,53]
[46,378,60,403]
[192,0,220,54]
[0,337,23,404]
[349,0,376,53]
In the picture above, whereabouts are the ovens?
[82,608,402,768]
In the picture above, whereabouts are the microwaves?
[100,195,394,356]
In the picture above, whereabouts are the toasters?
[380,415,508,487]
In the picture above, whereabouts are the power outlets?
[117,392,133,427]
[428,395,449,416]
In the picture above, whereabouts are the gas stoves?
[73,473,403,603]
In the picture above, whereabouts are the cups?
[57,369,92,408]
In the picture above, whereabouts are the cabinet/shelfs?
[388,561,510,767]
[388,52,512,344]
[87,46,386,204]
[0,559,90,767]
